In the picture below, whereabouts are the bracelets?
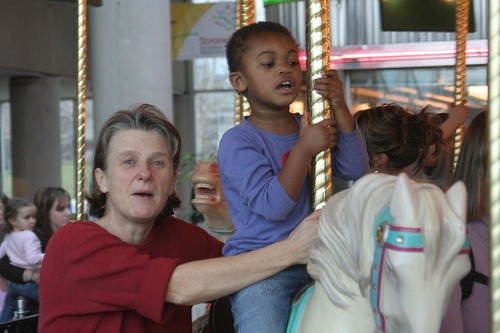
[30,270,36,281]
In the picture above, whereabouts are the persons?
[0,187,73,324]
[37,103,322,333]
[217,21,491,333]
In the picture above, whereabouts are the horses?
[286,171,470,333]
[191,161,236,242]
